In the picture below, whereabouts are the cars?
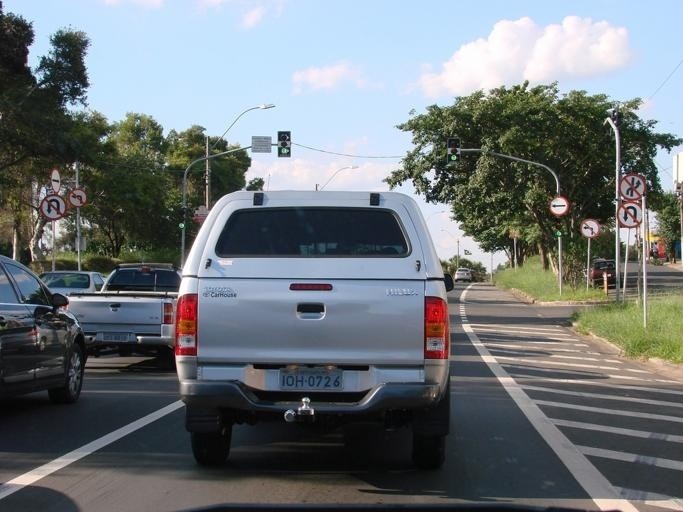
[175,188,453,468]
[0,255,88,404]
[455,267,472,283]
[590,259,623,289]
[36,270,104,298]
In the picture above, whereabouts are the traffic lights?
[447,138,461,163]
[554,217,563,237]
[278,132,291,156]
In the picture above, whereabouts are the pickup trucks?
[66,262,182,368]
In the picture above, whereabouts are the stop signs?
[550,196,570,215]
[51,169,61,193]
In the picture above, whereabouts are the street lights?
[316,165,359,191]
[440,229,459,268]
[205,104,276,210]
